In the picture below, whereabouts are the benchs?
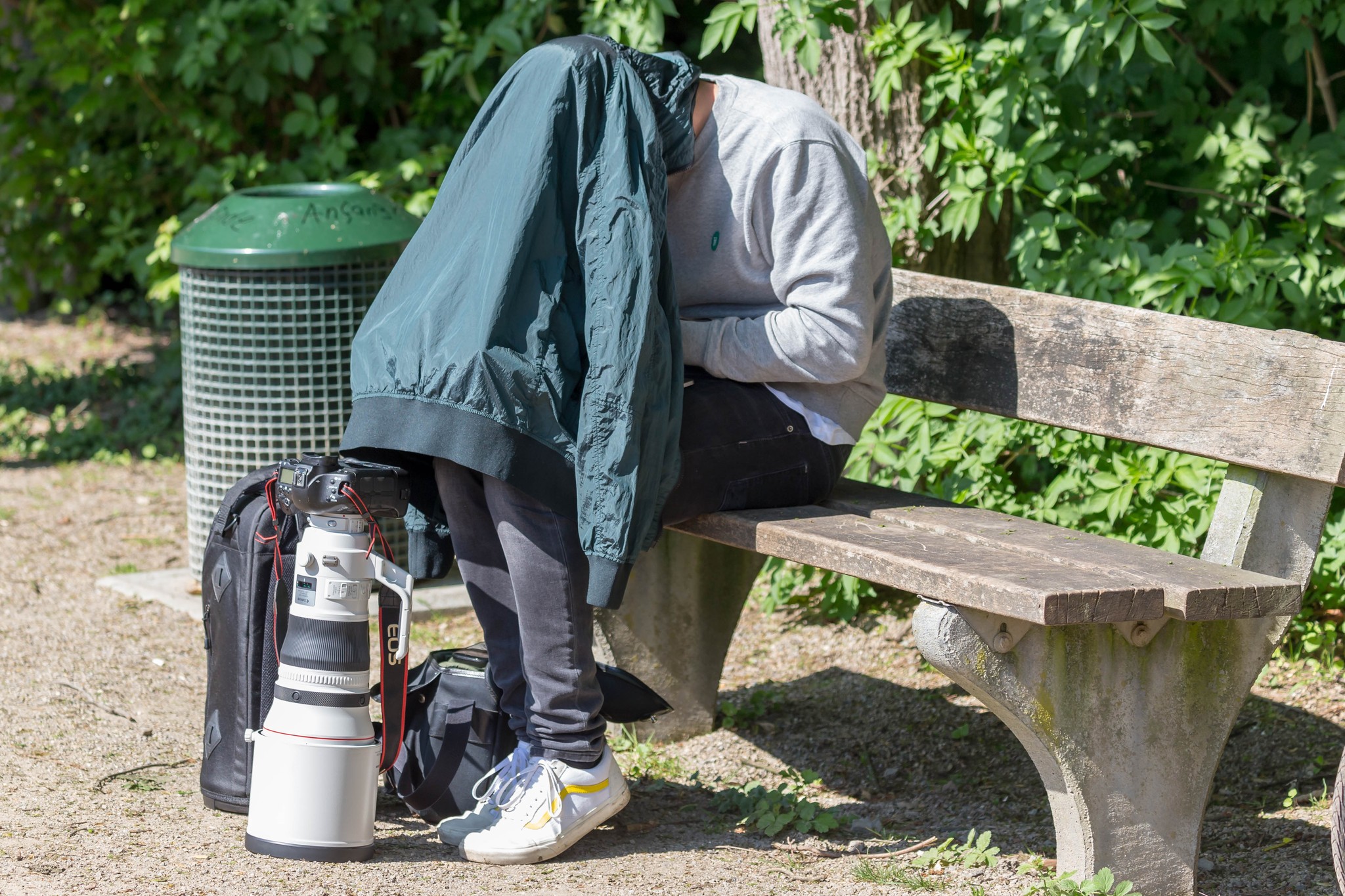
[594,266,1345,896]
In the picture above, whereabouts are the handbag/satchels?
[368,643,674,827]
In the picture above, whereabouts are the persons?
[340,35,895,863]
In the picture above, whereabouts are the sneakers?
[457,743,629,866]
[436,740,534,847]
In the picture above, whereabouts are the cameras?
[274,451,410,519]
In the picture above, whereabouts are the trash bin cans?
[170,182,427,596]
